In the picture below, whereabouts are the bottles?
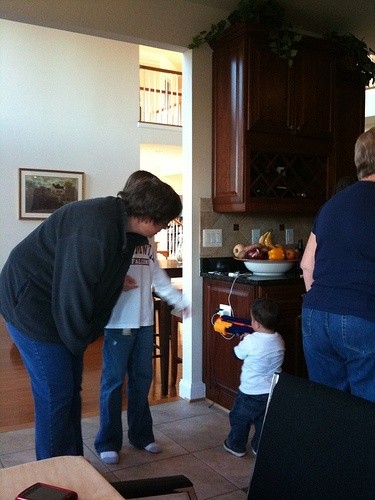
[298,240,304,256]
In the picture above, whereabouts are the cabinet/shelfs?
[208,21,365,215]
[203,279,306,411]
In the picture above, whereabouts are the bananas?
[259,230,274,248]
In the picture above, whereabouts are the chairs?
[112,372,375,500]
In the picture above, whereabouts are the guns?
[213,314,256,341]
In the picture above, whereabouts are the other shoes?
[223,440,247,457]
[252,448,257,454]
[100,451,119,464]
[142,442,160,453]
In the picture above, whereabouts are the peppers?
[244,244,285,260]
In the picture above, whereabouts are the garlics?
[285,248,295,260]
[232,243,244,257]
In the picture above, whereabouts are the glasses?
[160,223,170,229]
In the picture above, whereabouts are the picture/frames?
[18,168,86,220]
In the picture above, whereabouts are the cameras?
[15,482,79,500]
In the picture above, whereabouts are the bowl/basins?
[245,262,298,278]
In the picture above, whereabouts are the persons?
[300,127,375,401]
[223,298,285,456]
[0,178,183,460]
[94,170,190,462]
